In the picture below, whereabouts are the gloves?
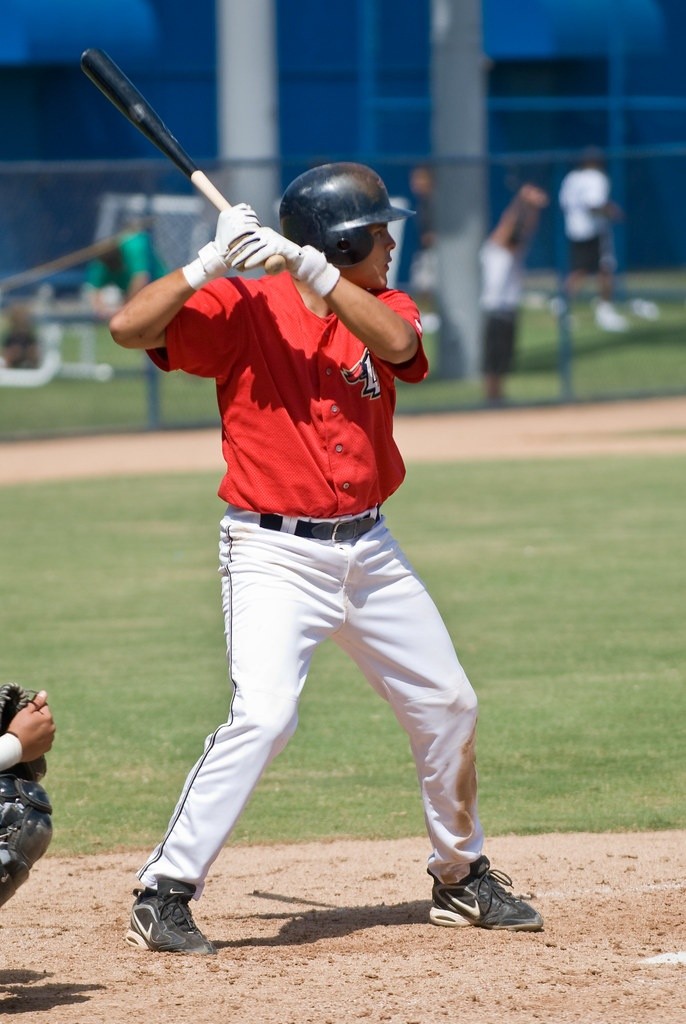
[182,203,262,291]
[224,228,341,297]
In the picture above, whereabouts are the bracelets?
[0,732,23,772]
[180,258,209,290]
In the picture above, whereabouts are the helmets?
[280,163,419,267]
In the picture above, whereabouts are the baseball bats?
[80,45,285,275]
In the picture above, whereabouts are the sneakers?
[427,855,544,930]
[125,878,218,959]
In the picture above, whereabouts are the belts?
[260,504,382,541]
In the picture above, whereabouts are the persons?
[107,160,544,956]
[0,690,55,910]
[0,153,628,403]
